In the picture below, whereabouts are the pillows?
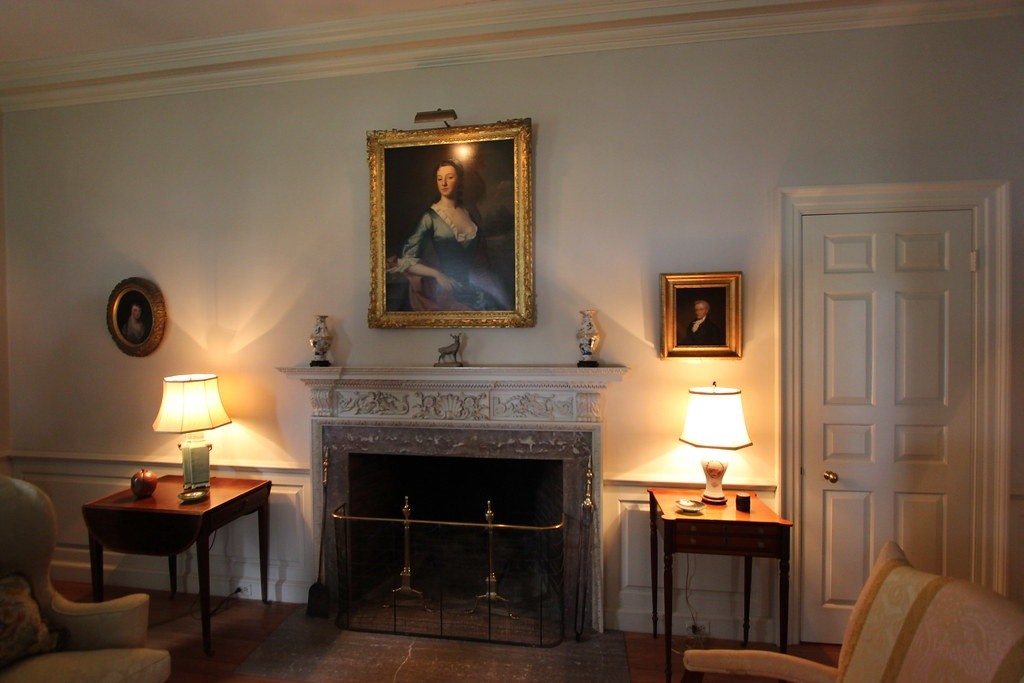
[0,575,59,667]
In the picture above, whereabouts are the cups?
[736,492,752,512]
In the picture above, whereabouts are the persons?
[687,300,720,345]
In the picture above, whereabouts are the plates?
[178,489,210,500]
[674,500,707,512]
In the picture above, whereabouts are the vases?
[310,315,331,361]
[574,310,600,362]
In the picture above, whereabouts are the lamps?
[414,109,457,126]
[152,374,233,489]
[679,381,752,505]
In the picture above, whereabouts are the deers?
[438,333,461,363]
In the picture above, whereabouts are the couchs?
[0,474,172,683]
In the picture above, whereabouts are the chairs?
[682,540,1024,683]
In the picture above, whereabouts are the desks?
[646,488,794,683]
[83,474,273,657]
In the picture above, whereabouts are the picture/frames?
[658,271,742,362]
[365,116,536,329]
[107,277,167,356]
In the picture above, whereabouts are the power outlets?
[235,583,251,596]
[685,621,712,638]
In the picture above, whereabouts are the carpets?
[226,605,632,682]
